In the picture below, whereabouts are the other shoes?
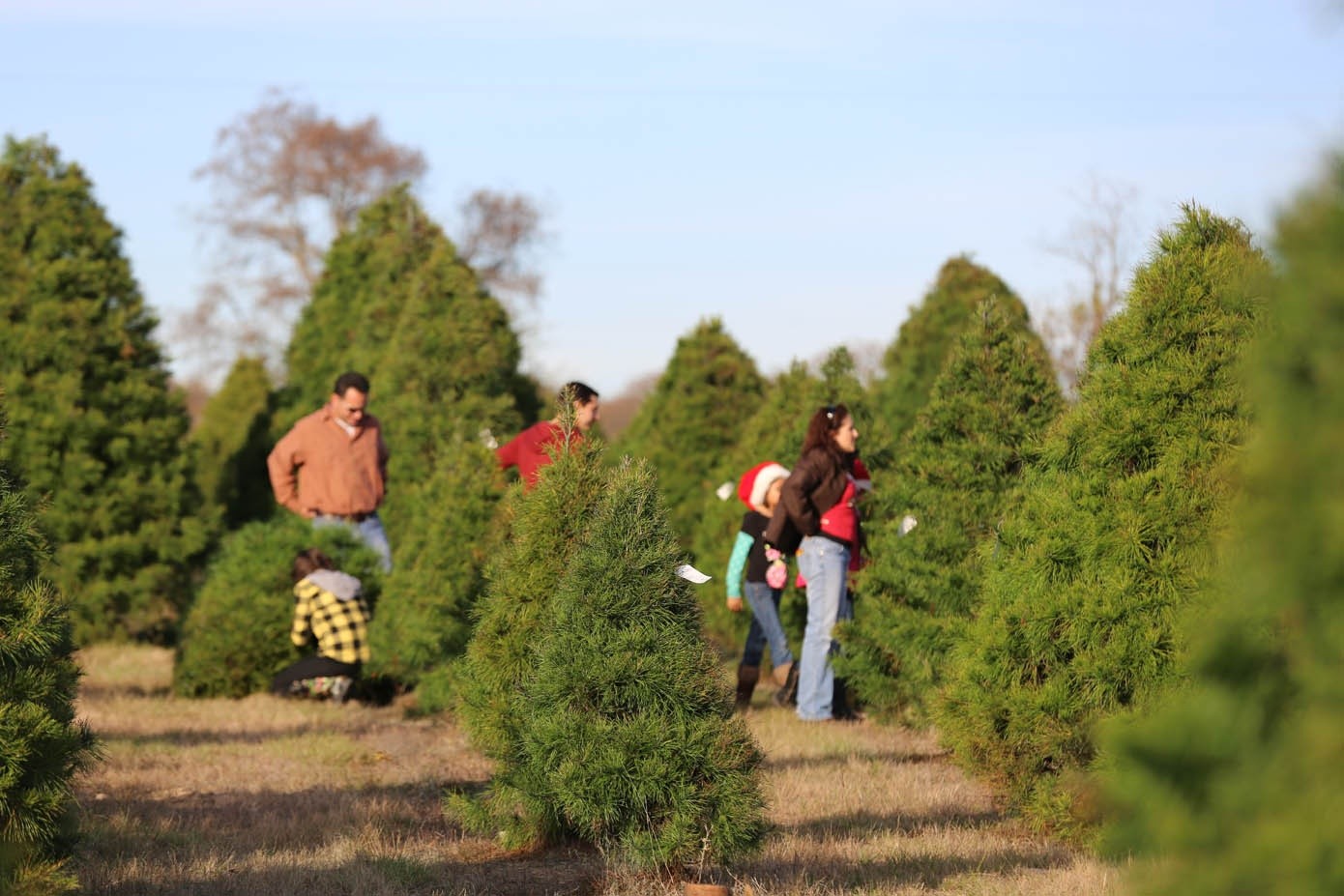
[330,675,353,704]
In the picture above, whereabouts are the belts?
[315,510,377,523]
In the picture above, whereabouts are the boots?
[733,665,762,714]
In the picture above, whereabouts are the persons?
[768,401,860,722]
[266,372,393,576]
[270,548,372,703]
[724,463,798,713]
[496,381,602,496]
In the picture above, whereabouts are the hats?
[740,462,791,515]
